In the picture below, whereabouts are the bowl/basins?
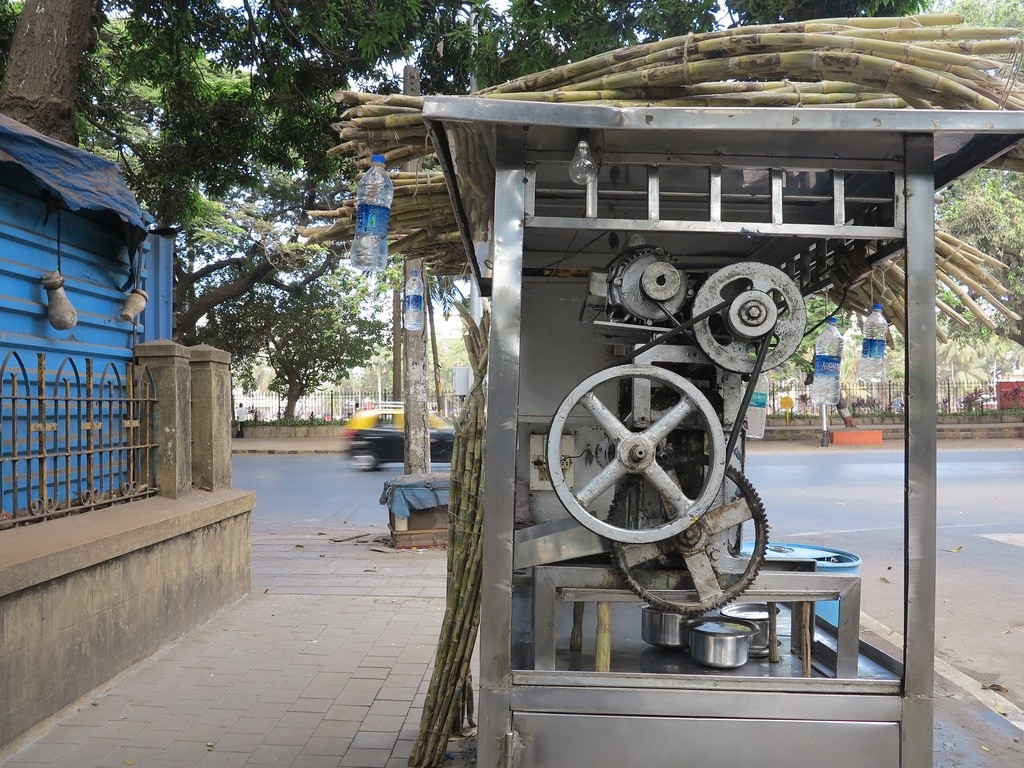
[637,604,705,648]
[717,601,771,650]
[678,617,761,668]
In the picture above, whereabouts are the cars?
[337,408,453,473]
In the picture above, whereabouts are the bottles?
[348,153,391,273]
[744,371,769,439]
[857,305,888,382]
[811,318,841,405]
[403,267,425,331]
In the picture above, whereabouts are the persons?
[236,403,247,437]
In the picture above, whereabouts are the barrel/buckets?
[740,540,861,630]
